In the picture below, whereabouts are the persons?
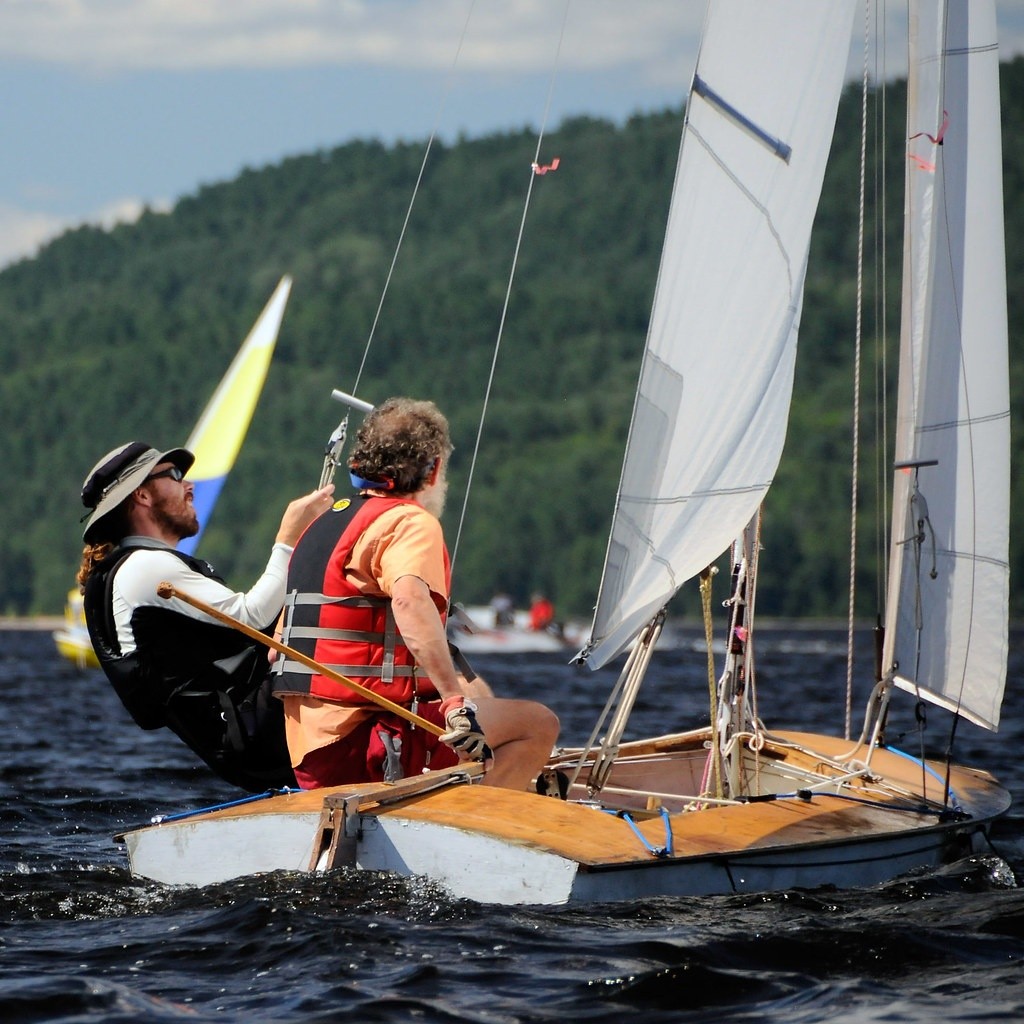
[530,591,554,630]
[268,397,561,791]
[78,441,335,794]
[489,592,514,626]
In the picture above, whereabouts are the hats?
[79,440,195,545]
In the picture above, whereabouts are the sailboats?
[112,0,1013,907]
[51,274,294,673]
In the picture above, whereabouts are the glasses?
[145,466,184,483]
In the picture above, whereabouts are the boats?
[449,604,680,657]
